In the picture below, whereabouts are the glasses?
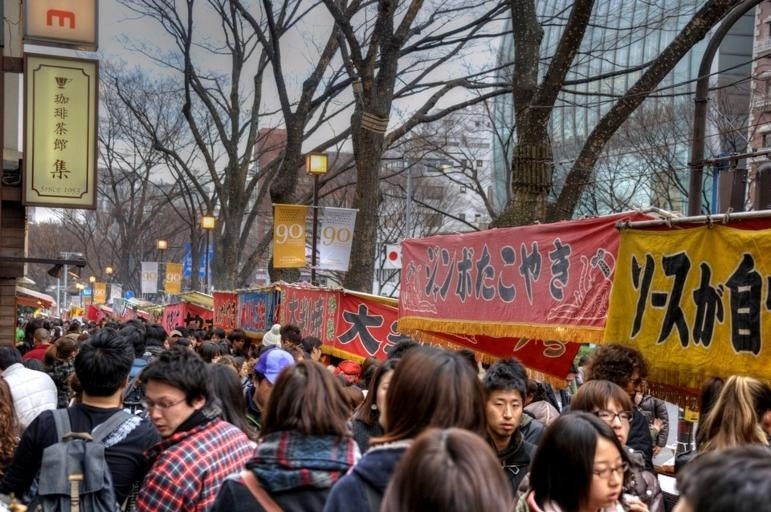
[592,409,633,423]
[140,395,188,408]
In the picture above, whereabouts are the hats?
[261,323,282,348]
[253,346,297,387]
[169,329,183,337]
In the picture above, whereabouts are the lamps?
[47,260,64,279]
[68,257,85,280]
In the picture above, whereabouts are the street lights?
[304,152,329,287]
[103,265,113,304]
[198,213,218,294]
[74,281,85,310]
[59,251,84,310]
[89,274,96,305]
[153,237,169,301]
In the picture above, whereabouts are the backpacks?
[33,405,136,512]
